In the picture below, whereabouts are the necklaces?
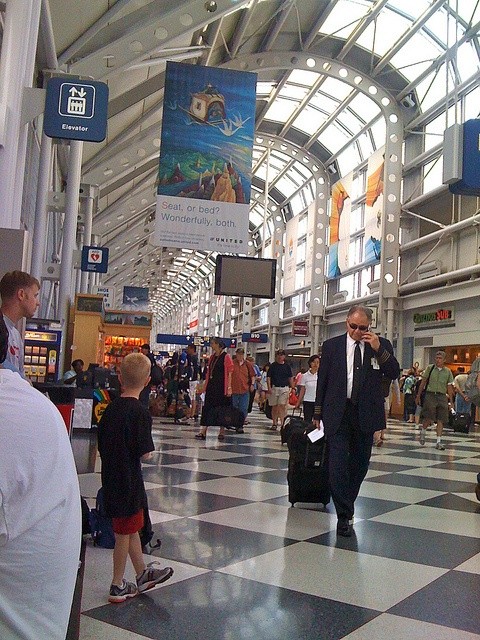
[210,350,224,380]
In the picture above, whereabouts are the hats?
[278,349,285,354]
[179,351,186,363]
[234,348,244,352]
[138,344,149,348]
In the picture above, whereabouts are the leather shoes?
[336,516,351,536]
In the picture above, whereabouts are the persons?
[0,269,45,372]
[414,371,437,430]
[445,382,455,425]
[294,369,305,406]
[196,336,234,440]
[177,354,195,423]
[201,357,208,378]
[0,314,82,640]
[165,351,181,412]
[402,367,415,420]
[309,306,400,538]
[297,354,320,420]
[244,358,262,425]
[187,346,201,421]
[415,351,454,450]
[140,341,155,413]
[267,348,297,427]
[452,364,471,422]
[466,354,480,501]
[60,359,84,389]
[259,361,270,409]
[97,351,174,604]
[231,348,256,434]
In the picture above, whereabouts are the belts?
[428,391,440,393]
[350,341,360,403]
[274,385,286,386]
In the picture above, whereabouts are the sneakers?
[236,427,242,432]
[108,578,136,602]
[414,424,419,429]
[136,561,172,592]
[435,442,444,449]
[420,439,424,444]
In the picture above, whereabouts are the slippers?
[194,432,205,438]
[406,420,412,422]
[270,425,276,429]
[217,434,223,439]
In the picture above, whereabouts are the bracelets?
[292,387,296,391]
[251,383,255,386]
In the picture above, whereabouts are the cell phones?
[367,324,372,336]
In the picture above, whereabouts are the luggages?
[453,400,470,433]
[279,408,302,444]
[286,423,329,506]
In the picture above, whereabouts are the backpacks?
[87,487,160,548]
[148,356,163,385]
[414,379,422,393]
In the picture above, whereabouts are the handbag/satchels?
[288,392,297,404]
[418,364,434,407]
